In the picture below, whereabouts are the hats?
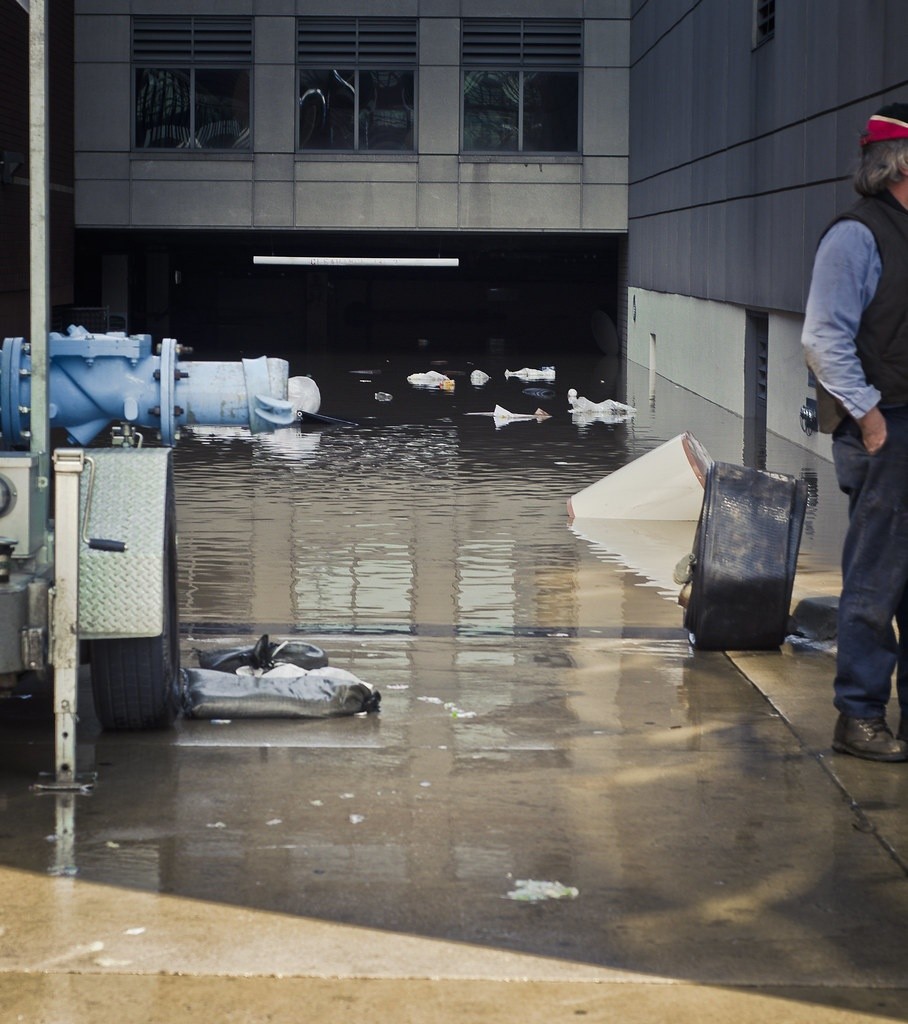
[860,103,908,146]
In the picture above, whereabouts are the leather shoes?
[832,712,908,761]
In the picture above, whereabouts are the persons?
[800,101,907,760]
